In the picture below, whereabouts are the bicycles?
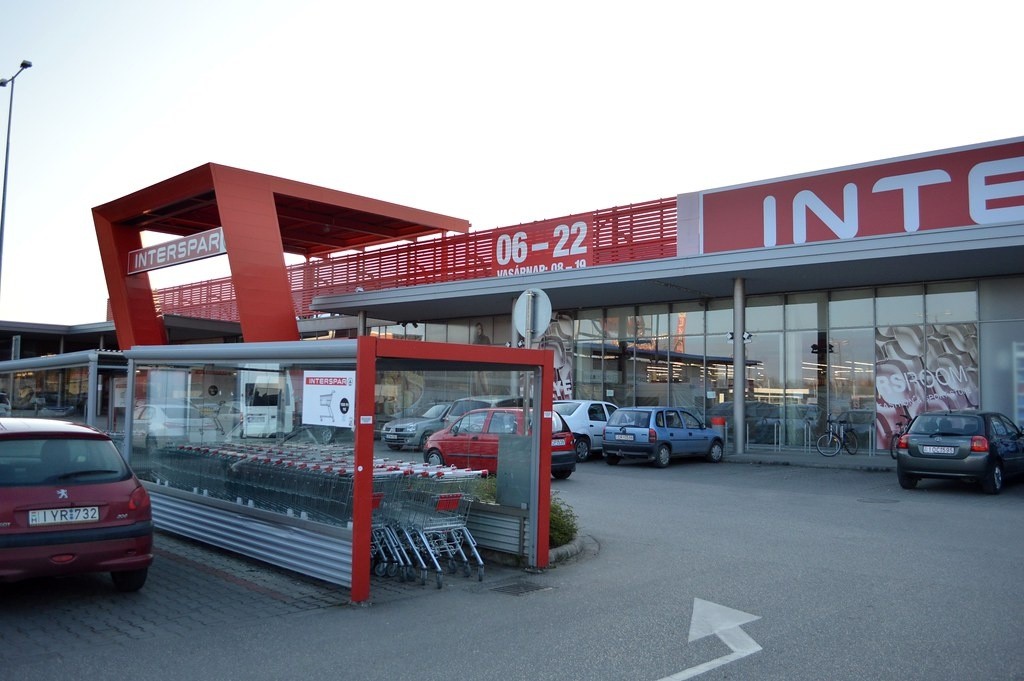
[890,415,913,460]
[815,413,859,457]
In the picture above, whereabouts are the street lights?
[0,60,34,284]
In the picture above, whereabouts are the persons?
[18,381,34,404]
[472,323,490,345]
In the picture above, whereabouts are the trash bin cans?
[711,416,726,442]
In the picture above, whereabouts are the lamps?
[355,287,364,293]
[401,323,408,327]
[412,323,418,328]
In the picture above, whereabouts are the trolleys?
[151,437,487,590]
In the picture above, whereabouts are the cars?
[422,406,577,480]
[0,364,352,459]
[827,409,875,446]
[698,400,778,441]
[380,401,452,453]
[0,417,152,593]
[601,405,723,469]
[552,400,641,462]
[896,410,1024,495]
[754,404,820,446]
[444,395,533,429]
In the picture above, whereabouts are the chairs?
[37,440,70,481]
[939,420,952,431]
[924,422,939,433]
[667,415,678,428]
[964,424,978,432]
